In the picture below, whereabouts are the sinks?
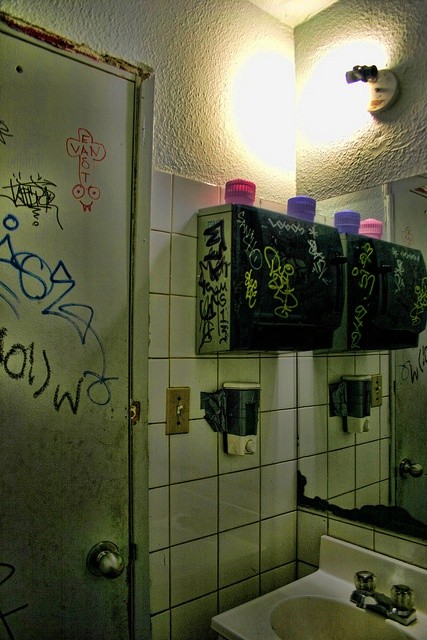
[270,595,415,640]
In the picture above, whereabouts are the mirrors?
[295,171,427,542]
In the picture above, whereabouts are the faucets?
[350,568,415,625]
